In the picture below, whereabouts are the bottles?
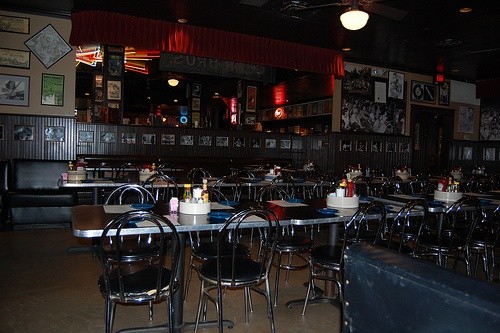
[202,190,209,204]
[198,198,204,203]
[143,163,157,172]
[344,164,362,181]
[183,184,192,202]
[67,161,86,172]
[336,179,356,197]
[155,106,163,125]
[438,179,460,193]
[190,197,198,203]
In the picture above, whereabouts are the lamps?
[342,36,353,52]
[340,0,369,30]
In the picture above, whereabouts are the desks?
[69,193,500,254]
[62,181,407,204]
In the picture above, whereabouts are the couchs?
[0,158,74,231]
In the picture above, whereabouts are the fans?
[288,0,409,22]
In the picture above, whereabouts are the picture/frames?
[0,15,229,146]
[233,70,474,153]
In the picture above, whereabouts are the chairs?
[94,162,500,333]
